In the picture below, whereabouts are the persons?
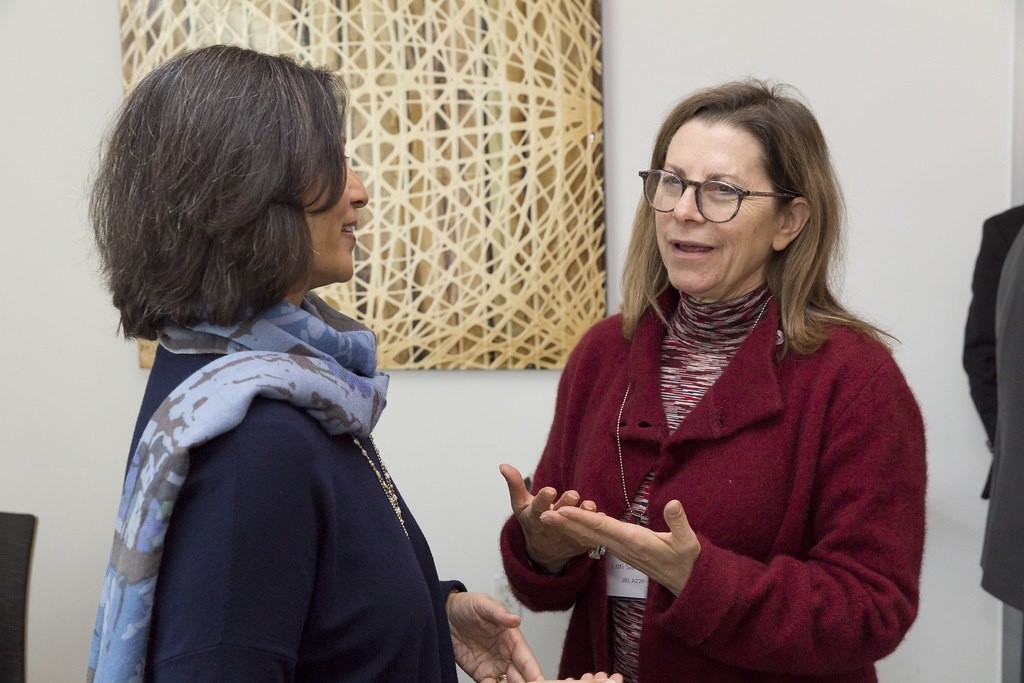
[88,46,632,683]
[963,205,1024,618]
[494,77,926,682]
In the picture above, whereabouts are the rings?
[595,544,606,555]
[496,674,507,682]
[588,548,601,559]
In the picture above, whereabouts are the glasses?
[639,169,795,225]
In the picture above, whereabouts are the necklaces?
[616,295,773,526]
[352,432,410,542]
